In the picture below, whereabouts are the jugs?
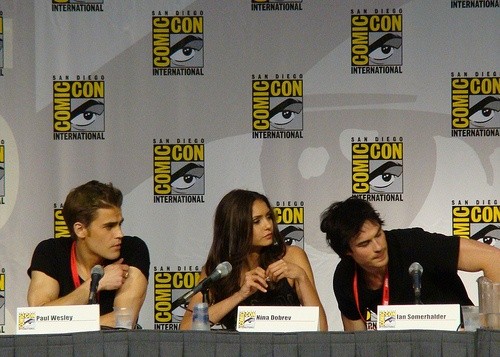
[476,276,500,331]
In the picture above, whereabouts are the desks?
[0,327,500,357]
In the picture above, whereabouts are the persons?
[26,181,150,331]
[180,189,329,331]
[319,195,500,331]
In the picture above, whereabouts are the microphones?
[409,262,424,301]
[88,265,105,305]
[177,261,233,308]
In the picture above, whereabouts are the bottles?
[192,304,210,331]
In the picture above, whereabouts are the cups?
[461,306,480,331]
[113,305,132,330]
